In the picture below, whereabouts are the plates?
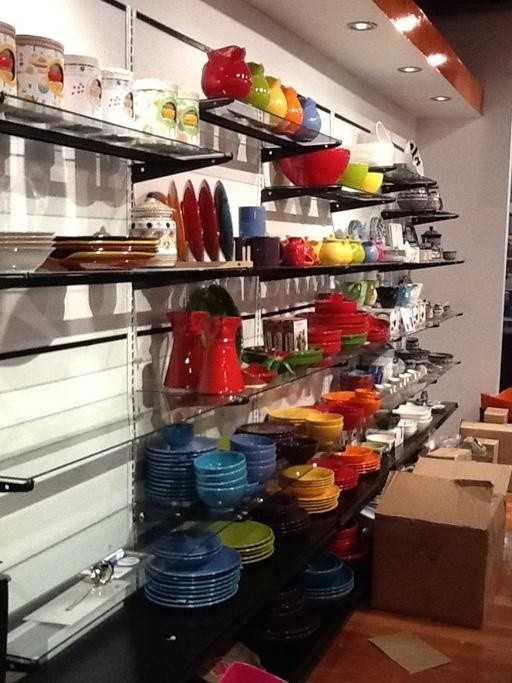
[144,447,381,608]
[241,312,390,387]
[405,223,418,247]
[144,438,220,506]
[166,177,233,261]
[372,120,424,178]
[349,218,386,245]
[56,235,159,271]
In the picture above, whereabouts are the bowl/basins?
[395,349,429,366]
[394,403,433,442]
[313,288,357,315]
[374,410,400,430]
[277,149,383,199]
[192,388,383,513]
[161,421,194,447]
[1,226,54,275]
[341,369,375,388]
[428,352,454,365]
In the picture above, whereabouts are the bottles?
[130,192,179,269]
[424,302,452,319]
[421,227,441,246]
[167,309,245,405]
[0,20,203,156]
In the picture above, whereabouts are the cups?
[376,363,427,408]
[365,433,396,454]
[443,251,456,260]
[234,207,305,269]
[407,337,419,353]
[343,281,400,309]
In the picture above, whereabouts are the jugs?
[202,43,321,144]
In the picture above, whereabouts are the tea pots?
[395,283,414,306]
[320,233,384,265]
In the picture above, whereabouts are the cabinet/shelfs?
[0,34,468,677]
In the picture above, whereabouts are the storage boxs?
[370,406,512,627]
